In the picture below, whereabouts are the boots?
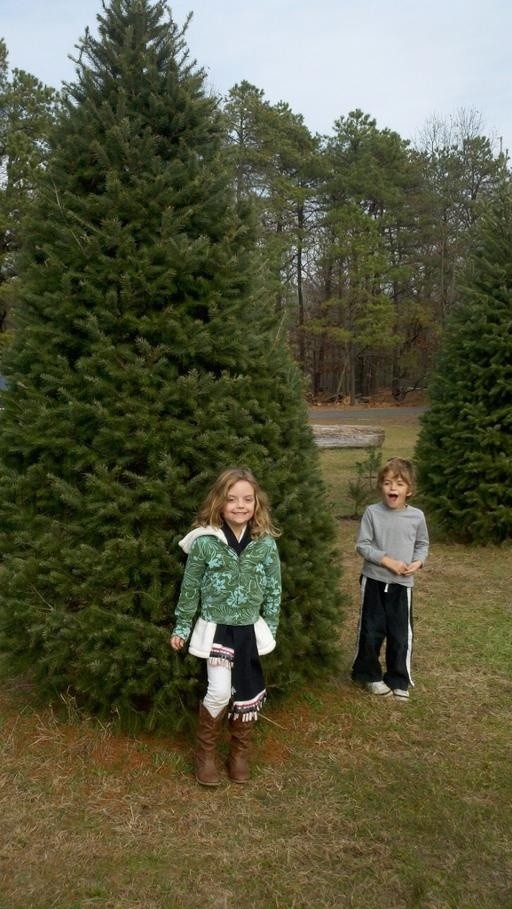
[227,713,252,786]
[194,699,229,787]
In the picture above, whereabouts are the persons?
[167,466,284,789]
[349,455,432,704]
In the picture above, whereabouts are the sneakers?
[393,688,411,701]
[365,679,394,698]
